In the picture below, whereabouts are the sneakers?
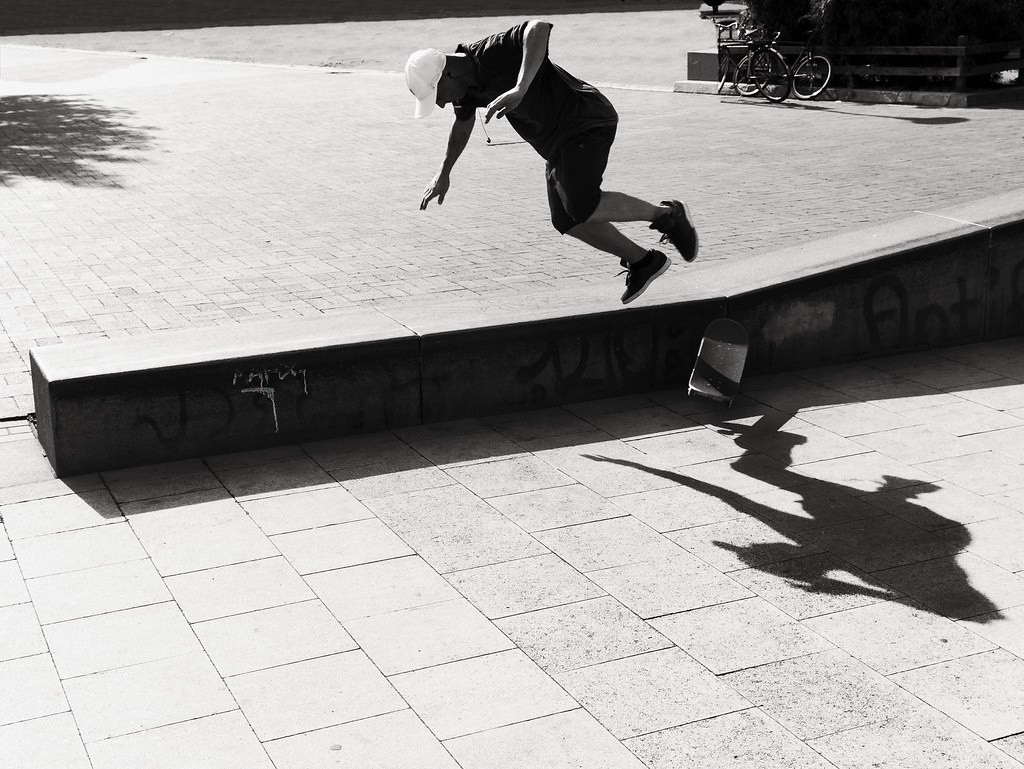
[621,248,671,304]
[649,198,699,262]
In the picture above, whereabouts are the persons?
[403,19,701,304]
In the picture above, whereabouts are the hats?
[405,47,446,118]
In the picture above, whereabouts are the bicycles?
[700,10,833,103]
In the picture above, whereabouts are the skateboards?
[687,318,749,410]
